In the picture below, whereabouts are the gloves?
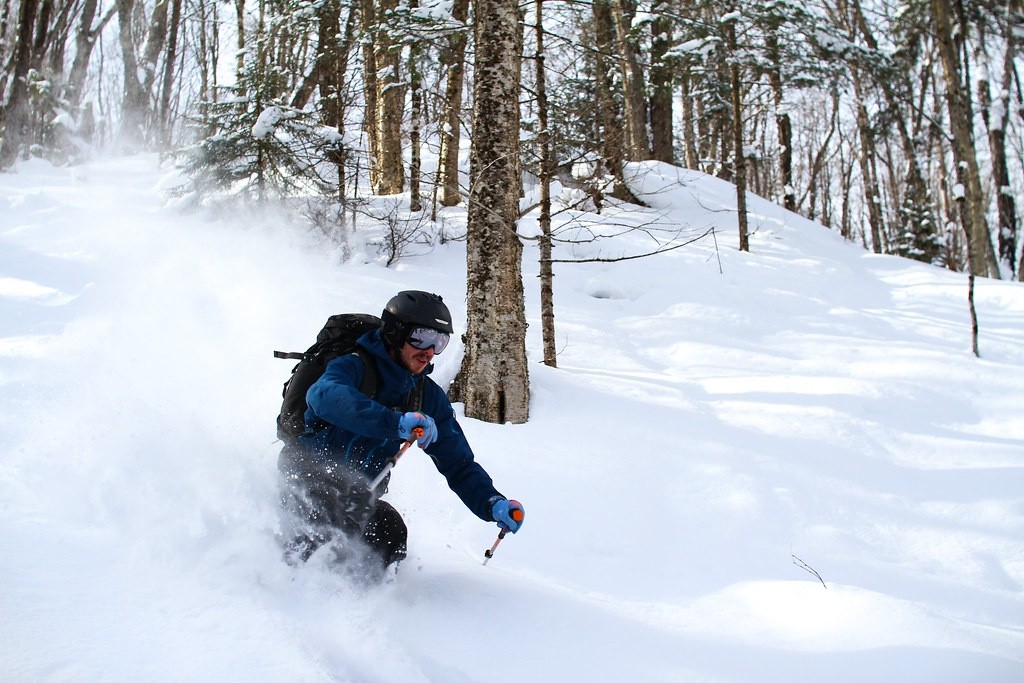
[398,410,438,449]
[491,499,525,534]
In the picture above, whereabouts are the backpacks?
[272,313,423,446]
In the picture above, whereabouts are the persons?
[278,289,524,589]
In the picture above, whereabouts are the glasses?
[403,322,450,355]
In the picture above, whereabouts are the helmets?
[380,290,453,350]
